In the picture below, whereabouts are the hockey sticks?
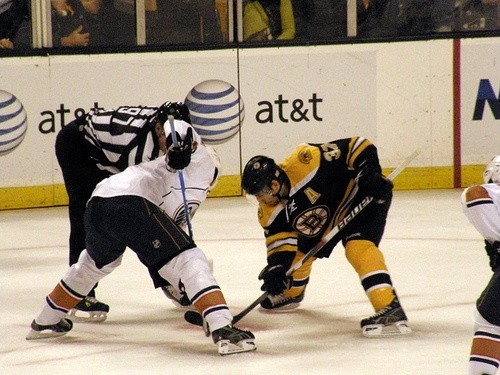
[168,115,210,337]
[184,147,422,327]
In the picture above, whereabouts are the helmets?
[160,100,191,124]
[204,144,220,180]
[240,155,275,196]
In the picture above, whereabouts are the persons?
[54,100,192,321]
[25,117,258,356]
[460,154,499,374]
[240,134,414,337]
[0,0,499,50]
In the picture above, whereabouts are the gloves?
[258,266,289,296]
[165,136,191,171]
[360,175,393,200]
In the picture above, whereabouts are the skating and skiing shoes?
[161,285,195,311]
[25,315,74,340]
[211,323,257,354]
[259,292,307,312]
[360,294,413,336]
[63,296,109,322]
